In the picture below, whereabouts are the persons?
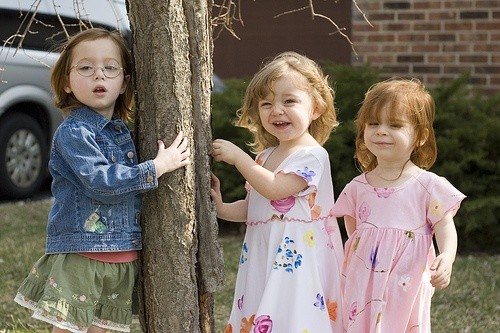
[210,52,344,333]
[330,76,467,333]
[13,28,191,333]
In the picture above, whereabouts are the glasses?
[67,61,130,79]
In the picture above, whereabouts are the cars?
[0,1,228,201]
